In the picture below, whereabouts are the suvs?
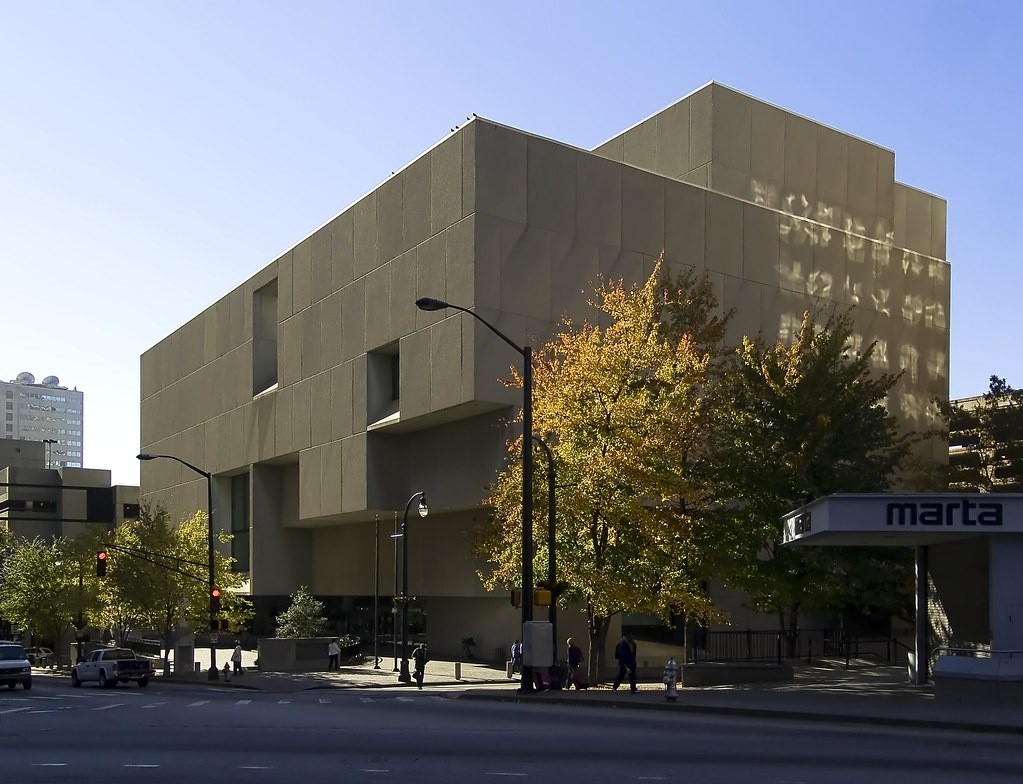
[0,644,34,691]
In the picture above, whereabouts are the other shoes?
[613,688,616,692]
[631,690,640,692]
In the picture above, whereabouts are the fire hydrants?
[221,662,231,682]
[662,656,685,703]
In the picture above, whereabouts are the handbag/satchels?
[577,647,584,663]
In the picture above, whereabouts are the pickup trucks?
[72,648,156,690]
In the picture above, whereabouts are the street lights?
[53,548,85,662]
[415,293,540,696]
[397,491,430,684]
[135,452,220,683]
[42,438,58,470]
[518,436,564,693]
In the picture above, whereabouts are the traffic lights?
[209,586,222,615]
[96,552,107,579]
[510,586,522,608]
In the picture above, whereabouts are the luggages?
[568,664,589,692]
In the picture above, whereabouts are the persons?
[231,639,243,676]
[612,633,641,694]
[511,640,521,675]
[411,643,429,690]
[561,638,584,691]
[328,639,339,672]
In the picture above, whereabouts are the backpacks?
[615,641,624,658]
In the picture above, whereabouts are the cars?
[24,647,54,663]
